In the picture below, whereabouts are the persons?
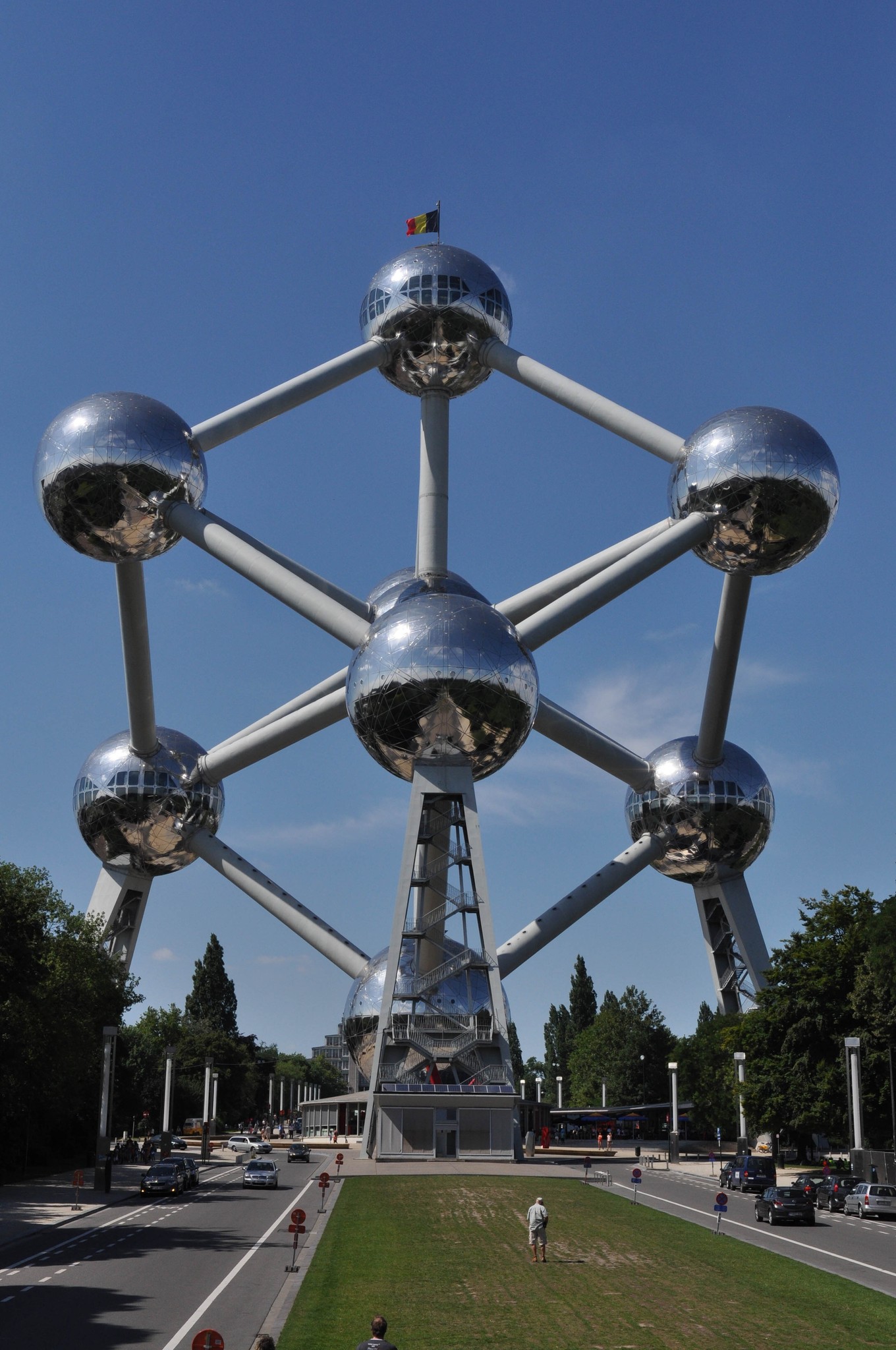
[528,1122,718,1151]
[112,1126,184,1166]
[239,1110,339,1144]
[817,1156,849,1170]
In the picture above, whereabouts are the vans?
[296,1118,302,1125]
[183,1118,204,1136]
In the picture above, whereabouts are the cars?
[288,1124,302,1134]
[160,1157,192,1190]
[187,1158,201,1187]
[149,1134,189,1150]
[242,1160,281,1189]
[754,1175,896,1224]
[287,1143,311,1164]
[140,1163,185,1197]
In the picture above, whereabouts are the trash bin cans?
[635,1146,640,1157]
[748,1149,752,1155]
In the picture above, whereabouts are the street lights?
[268,1073,276,1135]
[210,1073,219,1136]
[733,1052,748,1155]
[640,1054,647,1104]
[668,1062,681,1165]
[279,1076,286,1128]
[200,1056,216,1164]
[164,1047,174,1134]
[844,1037,864,1150]
[93,1026,118,1192]
[519,1076,606,1109]
[289,1078,295,1114]
[297,1080,321,1112]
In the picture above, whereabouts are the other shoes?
[541,1258,546,1262]
[532,1259,538,1262]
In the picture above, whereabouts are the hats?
[536,1198,544,1205]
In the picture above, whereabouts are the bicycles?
[757,1141,772,1154]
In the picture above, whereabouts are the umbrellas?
[580,1112,693,1141]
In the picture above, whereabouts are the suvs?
[228,1136,272,1155]
[718,1155,777,1193]
